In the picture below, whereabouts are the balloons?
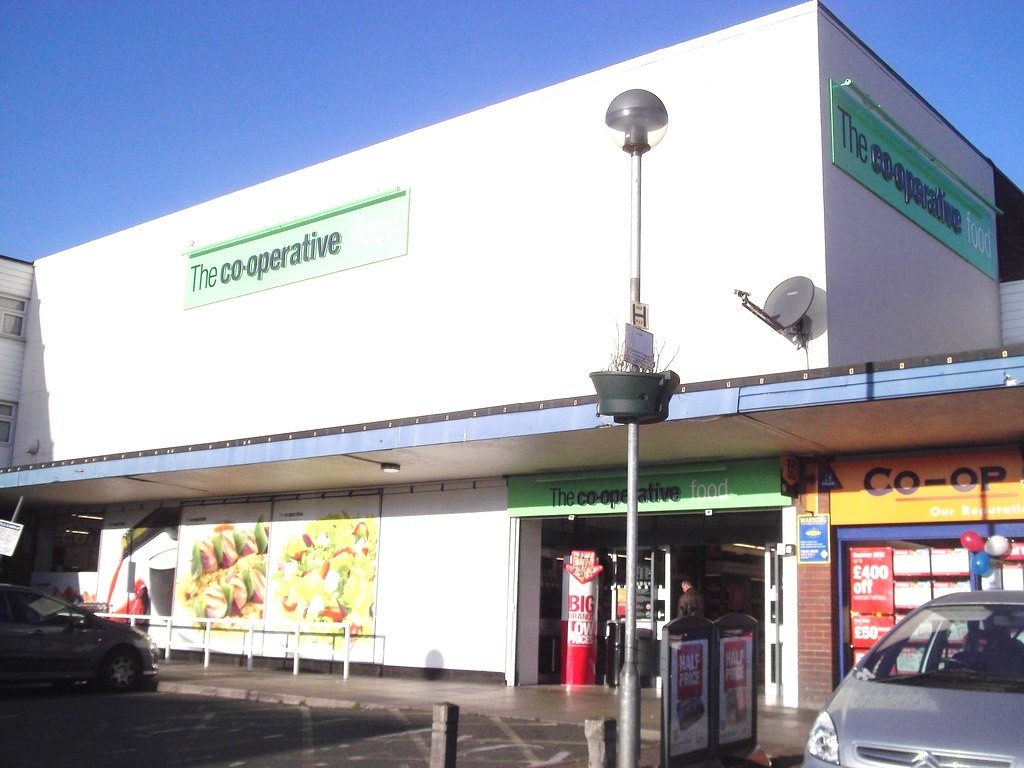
[961,530,985,553]
[971,551,989,575]
[983,535,1009,557]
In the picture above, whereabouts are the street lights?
[589,90,682,768]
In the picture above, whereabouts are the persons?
[678,579,705,624]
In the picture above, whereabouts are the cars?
[0,584,161,692]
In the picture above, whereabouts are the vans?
[804,589,1024,768]
